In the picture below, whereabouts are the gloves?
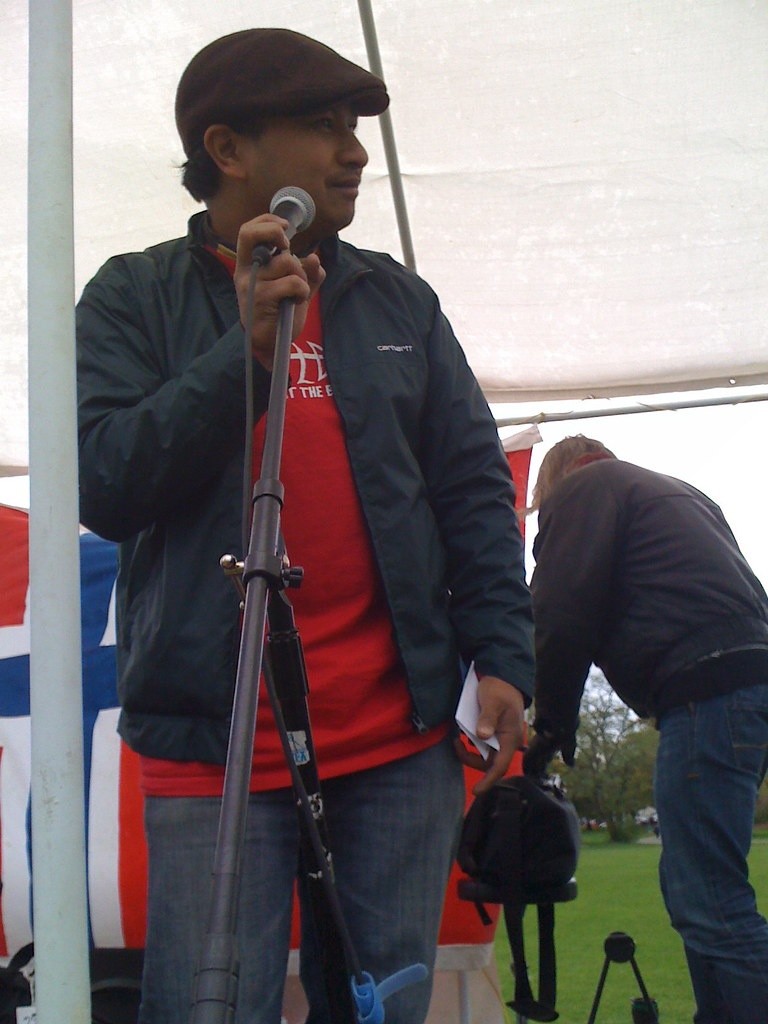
[523,720,579,786]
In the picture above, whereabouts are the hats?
[176,27,390,158]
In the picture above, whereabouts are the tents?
[5,0,767,1024]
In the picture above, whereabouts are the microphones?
[249,186,316,265]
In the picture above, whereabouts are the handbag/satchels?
[457,776,581,900]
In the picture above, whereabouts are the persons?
[529,434,767,1023]
[75,25,537,1023]
